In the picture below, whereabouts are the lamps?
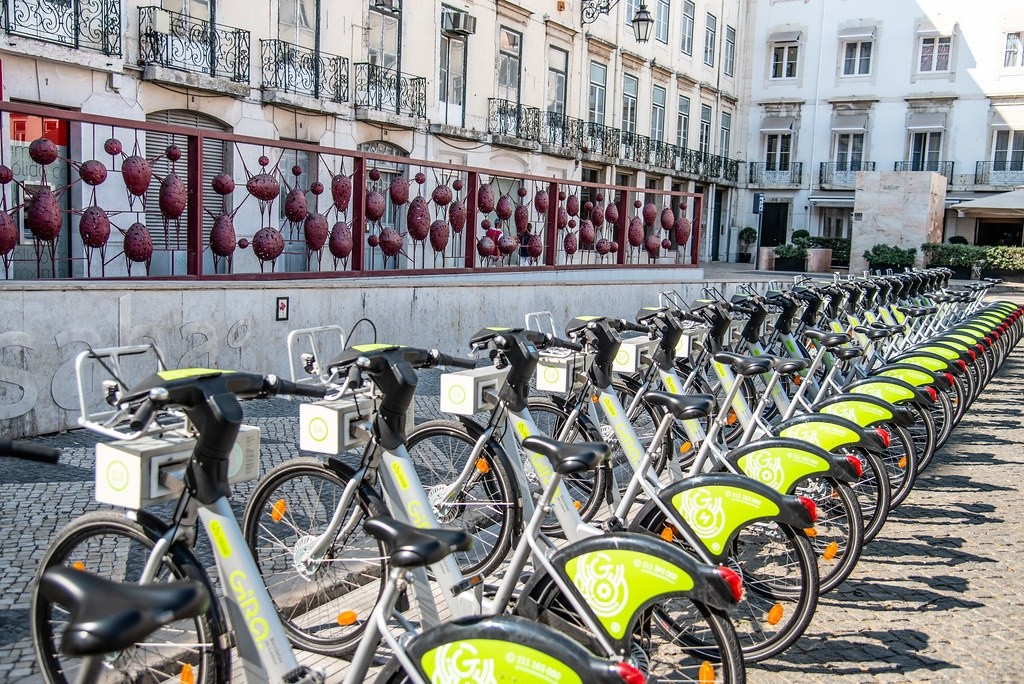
[581,0,654,44]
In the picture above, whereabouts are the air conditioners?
[446,13,477,36]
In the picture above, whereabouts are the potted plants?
[981,245,1024,283]
[791,229,809,249]
[920,243,991,281]
[862,243,917,276]
[738,227,757,263]
[774,244,809,272]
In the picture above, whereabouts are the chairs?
[972,264,982,278]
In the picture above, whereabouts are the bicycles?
[381,326,819,665]
[30,344,646,684]
[552,268,1024,555]
[483,311,864,601]
[243,318,747,684]
